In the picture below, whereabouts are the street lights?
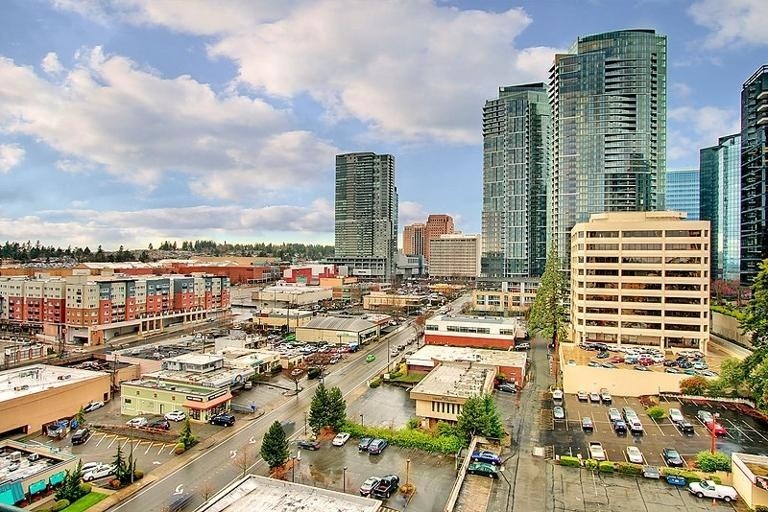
[406,456,411,484]
[359,415,365,427]
[304,410,308,436]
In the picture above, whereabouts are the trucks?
[687,477,739,502]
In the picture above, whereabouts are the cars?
[497,383,518,393]
[313,301,364,314]
[71,347,87,354]
[86,401,107,413]
[231,326,243,330]
[388,303,454,358]
[124,416,148,429]
[164,408,186,423]
[467,462,499,479]
[548,337,729,485]
[207,317,214,323]
[470,449,502,465]
[264,330,361,381]
[295,427,401,500]
[365,354,375,364]
[81,462,117,481]
[147,419,171,431]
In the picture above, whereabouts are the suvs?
[208,414,236,428]
[514,342,531,350]
[70,425,91,446]
